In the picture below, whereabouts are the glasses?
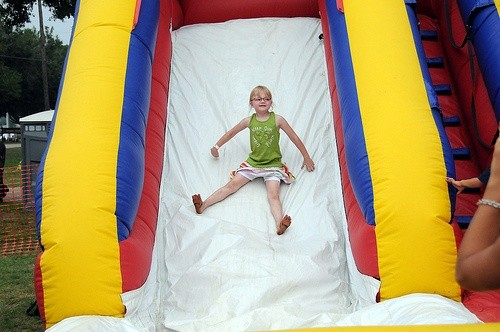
[250,97,271,102]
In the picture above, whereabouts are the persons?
[455,133,500,291]
[443,165,491,195]
[192,85,315,235]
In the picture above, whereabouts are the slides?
[36,1,457,332]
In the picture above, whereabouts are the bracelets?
[213,144,220,150]
[475,198,500,211]
